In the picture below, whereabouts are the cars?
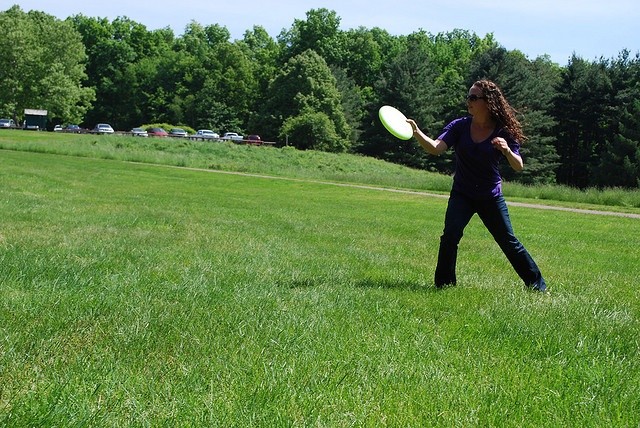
[244,135,260,141]
[146,127,169,136]
[170,129,188,137]
[54,125,63,131]
[196,130,219,138]
[224,132,243,140]
[94,124,114,134]
[0,119,16,128]
[131,128,148,137]
[62,125,85,134]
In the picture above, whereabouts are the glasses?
[467,93,484,102]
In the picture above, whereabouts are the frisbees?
[379,105,414,140]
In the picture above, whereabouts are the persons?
[405,80,548,294]
